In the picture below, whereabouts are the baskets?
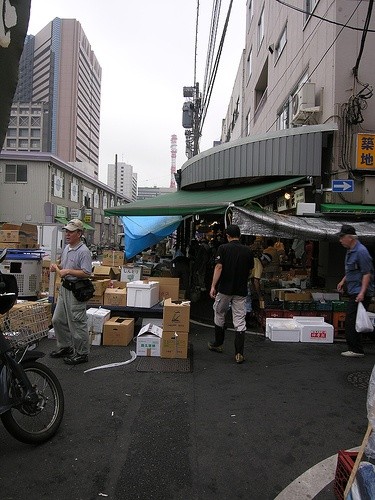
[287,299,345,312]
[0,298,51,347]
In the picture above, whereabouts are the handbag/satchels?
[72,279,96,303]
[355,302,374,333]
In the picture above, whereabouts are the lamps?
[284,186,295,200]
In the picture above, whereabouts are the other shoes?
[63,352,88,364]
[341,350,364,357]
[49,346,74,357]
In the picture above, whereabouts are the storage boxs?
[262,285,348,343]
[331,449,365,499]
[0,221,192,361]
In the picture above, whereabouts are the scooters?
[0,272,66,447]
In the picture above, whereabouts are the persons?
[336,225,372,355]
[208,224,254,363]
[50,218,92,364]
[187,224,272,320]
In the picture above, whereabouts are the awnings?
[122,216,183,260]
[102,174,305,216]
[320,204,375,214]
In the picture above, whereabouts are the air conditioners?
[290,82,317,123]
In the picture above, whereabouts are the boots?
[234,331,247,364]
[207,324,226,352]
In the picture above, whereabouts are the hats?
[336,224,357,236]
[62,219,83,232]
